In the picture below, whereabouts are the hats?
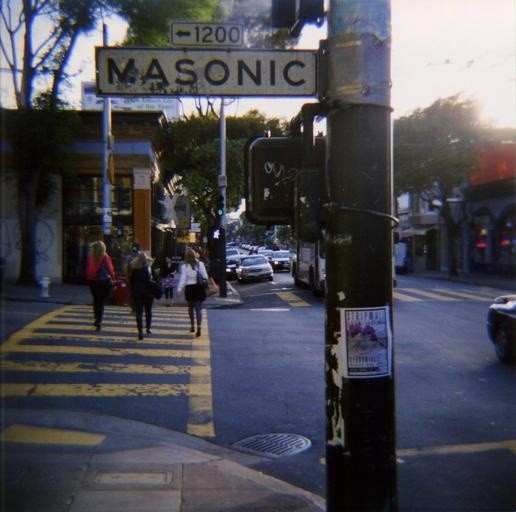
[224,238,293,283]
[486,293,516,361]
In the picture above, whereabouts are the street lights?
[197,271,218,301]
[149,282,163,299]
[161,279,176,287]
[97,255,114,290]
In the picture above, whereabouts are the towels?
[39,277,50,298]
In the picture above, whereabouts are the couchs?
[213,192,225,216]
[212,226,224,240]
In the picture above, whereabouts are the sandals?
[288,228,329,294]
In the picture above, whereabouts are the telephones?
[93,23,319,99]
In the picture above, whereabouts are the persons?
[124,244,147,314]
[177,249,210,337]
[160,255,176,307]
[86,240,118,330]
[129,253,156,340]
